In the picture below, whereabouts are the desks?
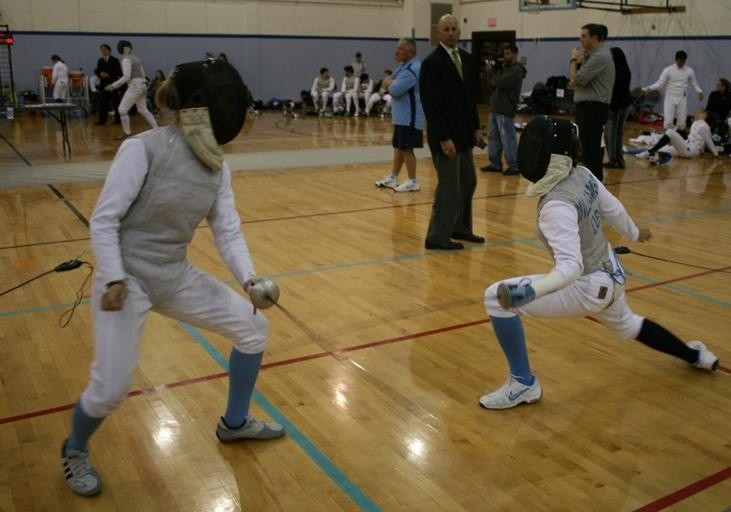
[0,102,76,158]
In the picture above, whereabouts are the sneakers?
[602,160,626,169]
[686,341,718,371]
[634,150,656,159]
[216,415,284,442]
[394,182,420,192]
[479,376,542,409]
[374,177,399,188]
[60,438,100,497]
[315,107,392,117]
[113,133,130,141]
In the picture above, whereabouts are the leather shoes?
[425,240,464,249]
[502,168,519,175]
[451,230,485,243]
[481,166,501,172]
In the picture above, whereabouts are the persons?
[49,54,69,106]
[638,50,731,165]
[59,59,284,497]
[577,23,610,71]
[150,68,166,112]
[475,118,719,410]
[418,13,490,251]
[375,33,424,194]
[89,67,104,115]
[479,43,529,178]
[568,23,615,183]
[529,81,553,115]
[92,43,124,125]
[102,40,161,141]
[310,52,394,118]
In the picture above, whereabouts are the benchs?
[299,85,394,116]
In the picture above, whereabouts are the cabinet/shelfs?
[39,71,89,109]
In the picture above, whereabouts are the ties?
[451,50,462,77]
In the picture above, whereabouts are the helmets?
[168,52,249,171]
[117,40,132,58]
[518,116,580,198]
